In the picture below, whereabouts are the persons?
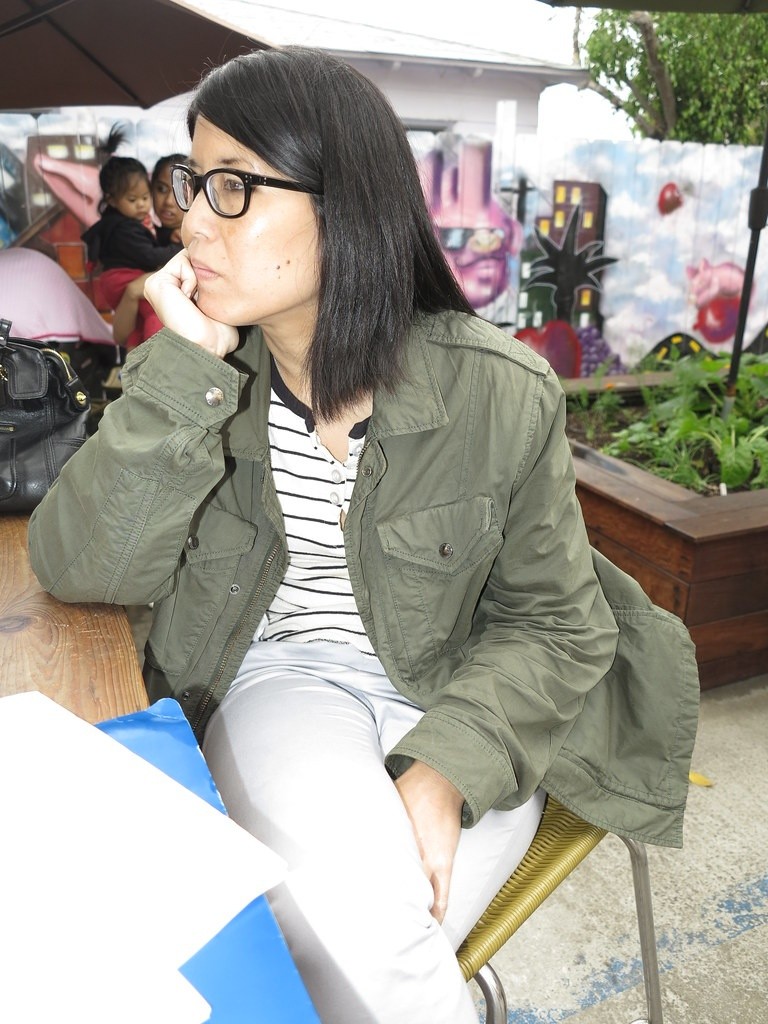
[78,119,185,272]
[24,45,703,1024]
[100,153,190,353]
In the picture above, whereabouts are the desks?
[0,512,323,1024]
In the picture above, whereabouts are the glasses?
[170,164,324,220]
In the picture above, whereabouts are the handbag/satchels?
[0,319,94,513]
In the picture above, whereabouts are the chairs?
[455,545,700,1024]
